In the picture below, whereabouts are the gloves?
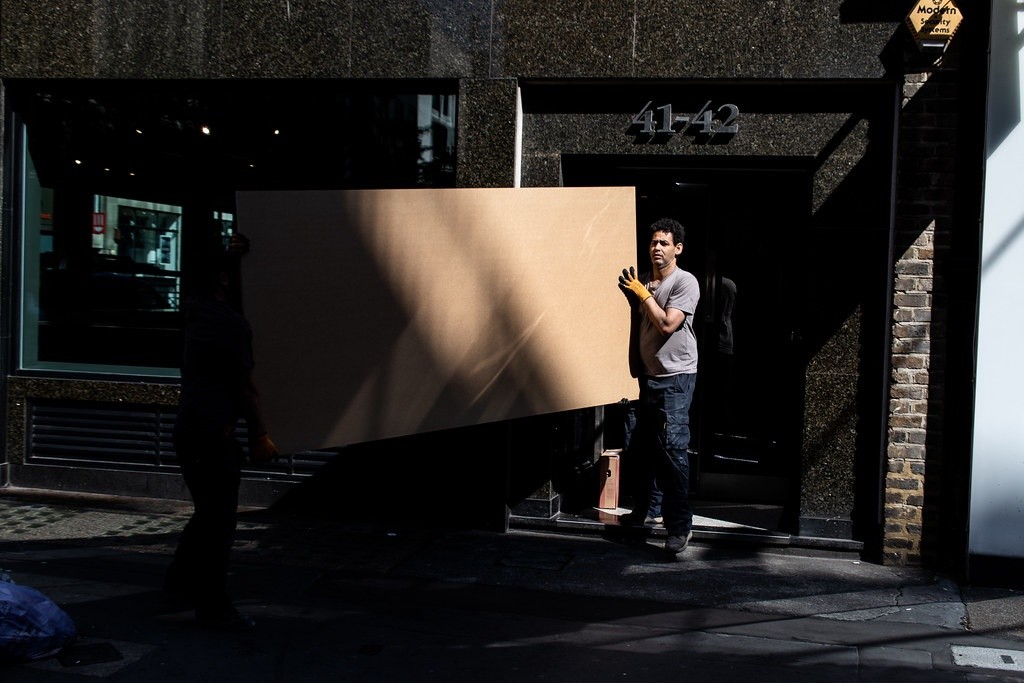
[226,234,254,258]
[254,434,280,460]
[618,266,653,305]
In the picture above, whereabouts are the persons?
[700,259,739,457]
[618,218,700,552]
[151,233,279,632]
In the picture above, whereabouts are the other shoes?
[195,603,252,632]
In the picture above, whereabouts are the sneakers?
[664,529,693,553]
[617,510,663,524]
